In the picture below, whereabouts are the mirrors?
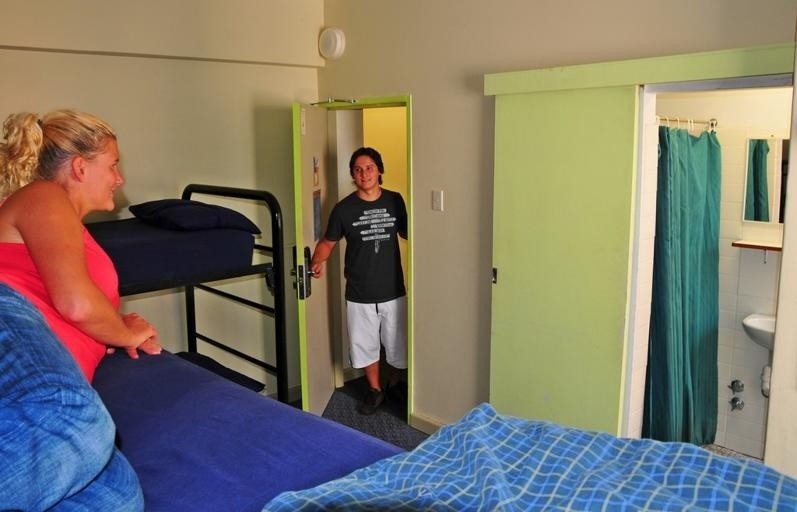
[742,133,790,225]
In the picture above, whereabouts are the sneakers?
[359,387,385,415]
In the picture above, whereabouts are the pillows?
[123,197,264,237]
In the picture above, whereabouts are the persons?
[308,147,407,415]
[0,107,164,511]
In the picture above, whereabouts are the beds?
[83,176,289,407]
[90,338,796,512]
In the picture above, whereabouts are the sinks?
[743,312,775,352]
